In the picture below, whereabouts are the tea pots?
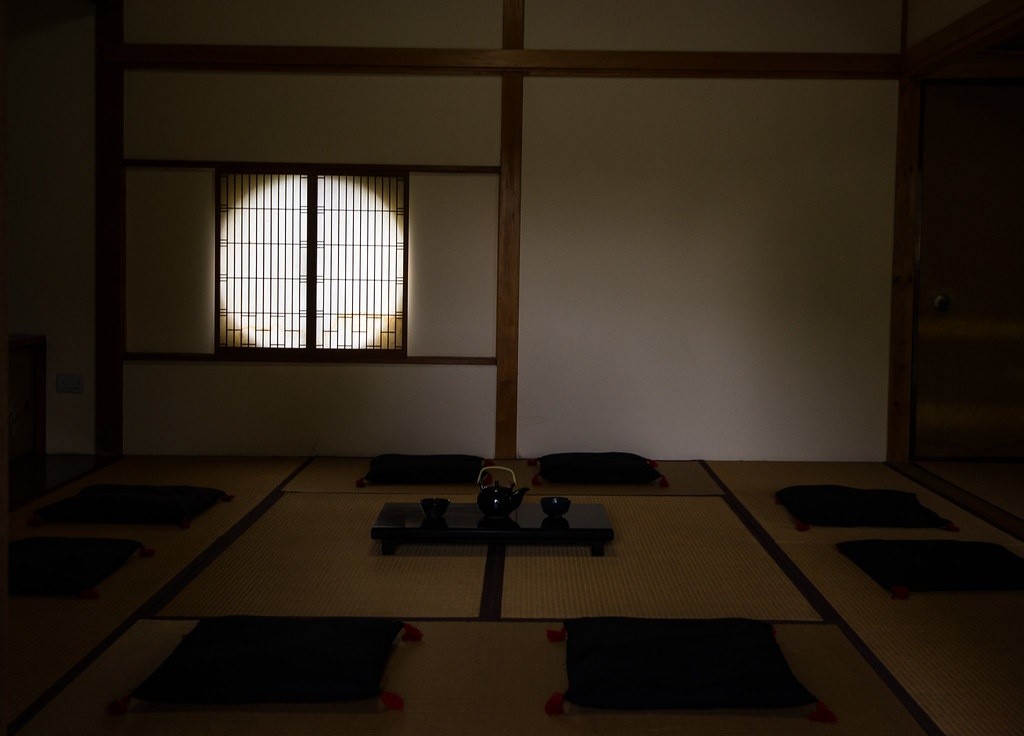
[477,466,529,518]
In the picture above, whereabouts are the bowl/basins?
[540,496,571,518]
[418,497,451,519]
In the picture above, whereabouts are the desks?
[370,503,613,557]
